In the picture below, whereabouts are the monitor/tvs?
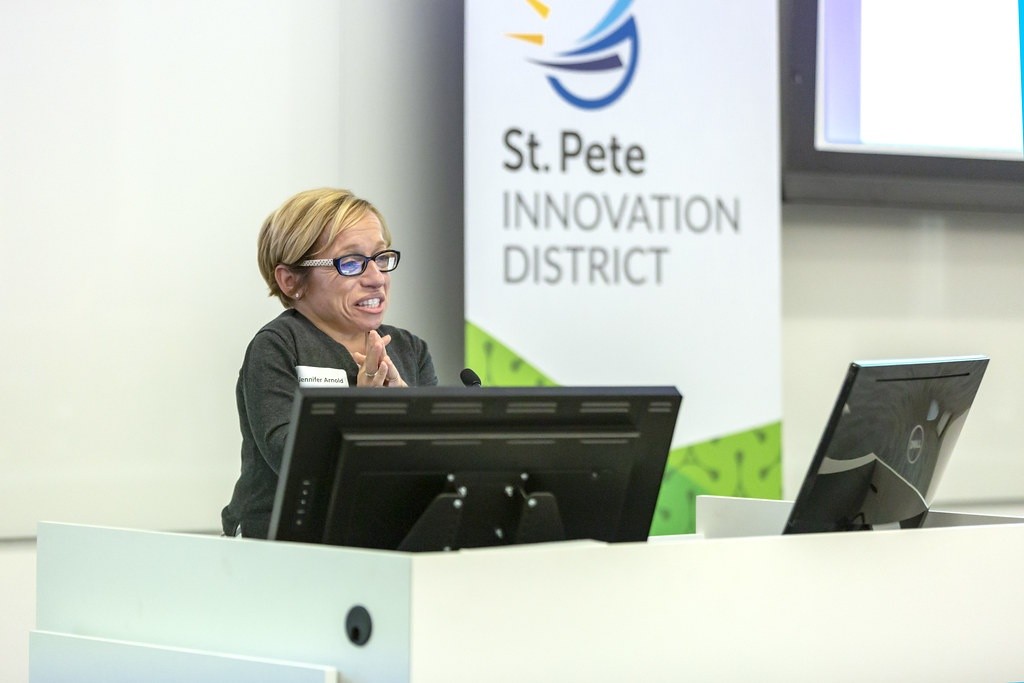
[783,356,991,536]
[268,384,682,551]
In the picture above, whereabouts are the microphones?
[459,369,482,386]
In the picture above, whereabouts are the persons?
[221,187,437,540]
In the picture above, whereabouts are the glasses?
[296,249,398,275]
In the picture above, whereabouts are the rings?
[364,370,379,377]
[386,373,399,382]
